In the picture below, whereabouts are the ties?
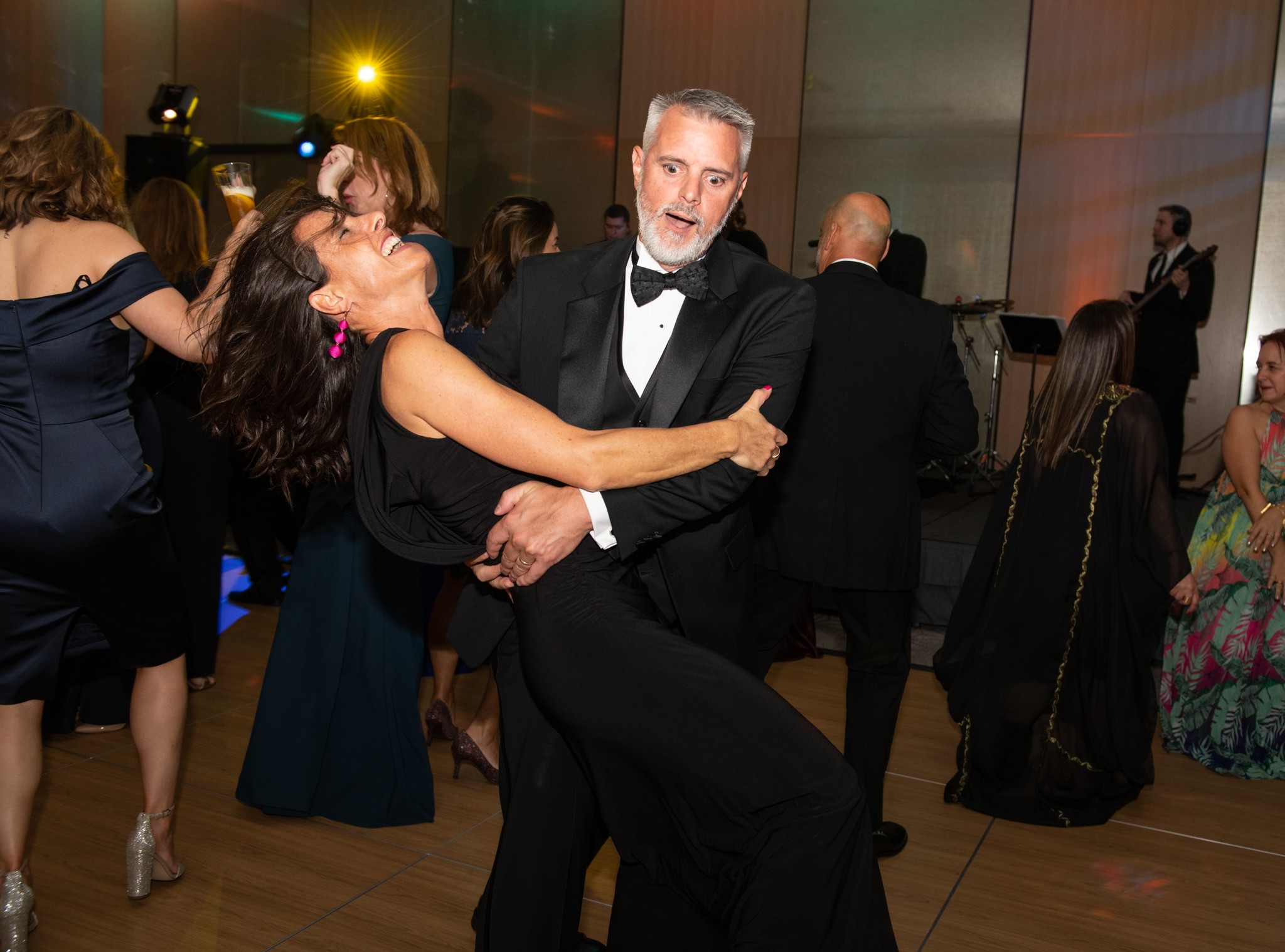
[1153,254,1168,285]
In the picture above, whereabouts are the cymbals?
[945,296,1016,318]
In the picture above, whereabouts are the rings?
[518,556,534,566]
[1182,595,1192,603]
[1275,580,1284,584]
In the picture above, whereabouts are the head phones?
[1171,204,1189,237]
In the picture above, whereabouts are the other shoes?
[228,585,286,606]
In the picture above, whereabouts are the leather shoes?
[872,821,910,858]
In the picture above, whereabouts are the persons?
[197,187,898,952]
[0,104,268,952]
[100,116,984,853]
[1158,330,1285,780]
[925,301,1203,831]
[430,82,824,951]
[1108,204,1215,496]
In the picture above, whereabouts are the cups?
[214,162,256,232]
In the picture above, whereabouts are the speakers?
[124,133,188,212]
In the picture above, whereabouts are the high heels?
[422,699,460,746]
[123,799,186,900]
[188,677,215,690]
[0,857,38,952]
[449,729,500,785]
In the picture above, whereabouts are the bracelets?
[1260,502,1284,525]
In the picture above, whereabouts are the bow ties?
[631,259,710,308]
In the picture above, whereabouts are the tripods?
[908,303,997,494]
[963,299,1013,482]
